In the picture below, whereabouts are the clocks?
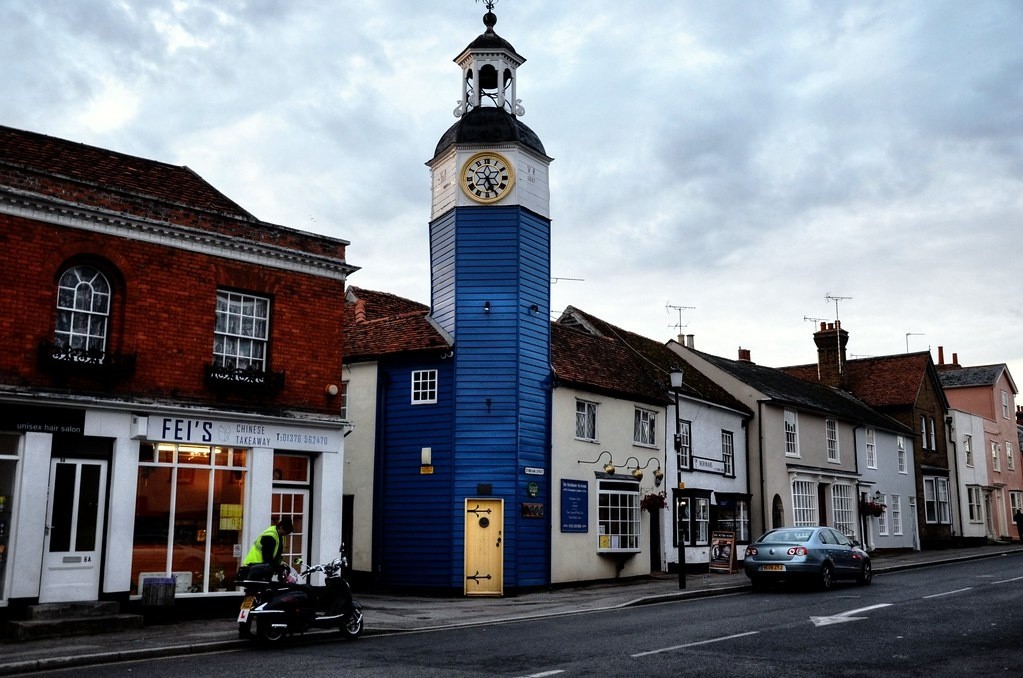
[460,152,514,203]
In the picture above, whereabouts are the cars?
[741,526,873,593]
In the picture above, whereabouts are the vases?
[873,513,881,517]
[214,587,227,591]
[648,504,659,513]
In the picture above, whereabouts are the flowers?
[187,568,227,593]
[640,493,669,512]
[860,499,889,517]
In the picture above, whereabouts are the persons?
[238,519,295,639]
[1013,510,1023,545]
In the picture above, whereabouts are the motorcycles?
[232,557,364,648]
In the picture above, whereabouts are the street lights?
[668,361,685,591]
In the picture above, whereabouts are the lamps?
[627,458,663,481]
[872,491,881,500]
[614,457,643,482]
[578,451,615,477]
